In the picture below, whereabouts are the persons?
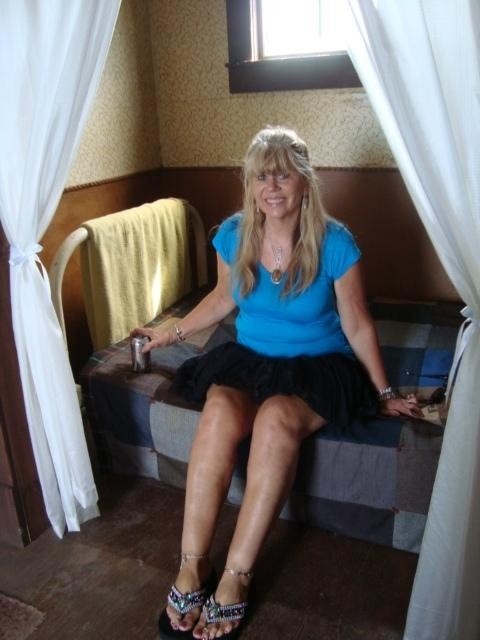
[131,127,424,640]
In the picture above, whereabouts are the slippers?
[158,583,209,640]
[190,592,251,640]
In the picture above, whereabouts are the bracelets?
[174,323,186,342]
[379,386,396,401]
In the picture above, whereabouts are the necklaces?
[264,220,292,284]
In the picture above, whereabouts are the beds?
[47,198,469,554]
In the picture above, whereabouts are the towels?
[80,195,193,353]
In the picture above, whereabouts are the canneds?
[130,335,151,373]
